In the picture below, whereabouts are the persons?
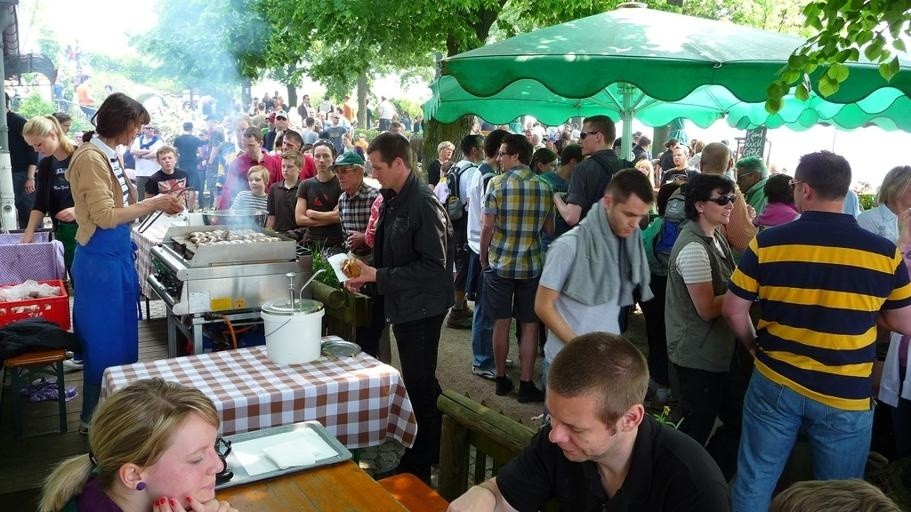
[448,333,733,511]
[56,76,109,119]
[665,175,737,448]
[720,153,909,509]
[534,152,653,394]
[770,478,901,511]
[5,92,44,227]
[64,91,185,434]
[39,377,236,511]
[173,91,462,440]
[22,114,83,368]
[446,115,634,402]
[633,133,911,485]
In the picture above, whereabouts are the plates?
[321,340,362,358]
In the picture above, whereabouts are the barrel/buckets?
[259,297,326,365]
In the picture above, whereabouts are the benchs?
[377,471,451,511]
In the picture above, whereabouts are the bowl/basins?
[203,209,268,232]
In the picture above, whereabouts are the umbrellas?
[429,1,911,168]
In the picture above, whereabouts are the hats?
[267,109,289,120]
[328,151,366,172]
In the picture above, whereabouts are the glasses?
[708,194,738,205]
[788,179,816,191]
[737,172,753,184]
[498,151,517,158]
[580,130,599,141]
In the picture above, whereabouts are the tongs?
[137,180,195,234]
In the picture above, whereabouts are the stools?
[4,345,72,438]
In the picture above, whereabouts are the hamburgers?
[342,258,361,278]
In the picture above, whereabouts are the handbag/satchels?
[56,221,79,266]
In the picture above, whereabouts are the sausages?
[189,229,281,246]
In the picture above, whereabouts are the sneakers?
[447,299,474,329]
[472,364,511,380]
[519,386,545,403]
[64,357,85,370]
[496,378,513,395]
[26,377,76,402]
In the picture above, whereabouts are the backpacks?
[655,175,732,269]
[434,161,468,244]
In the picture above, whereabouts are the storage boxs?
[0,231,66,284]
